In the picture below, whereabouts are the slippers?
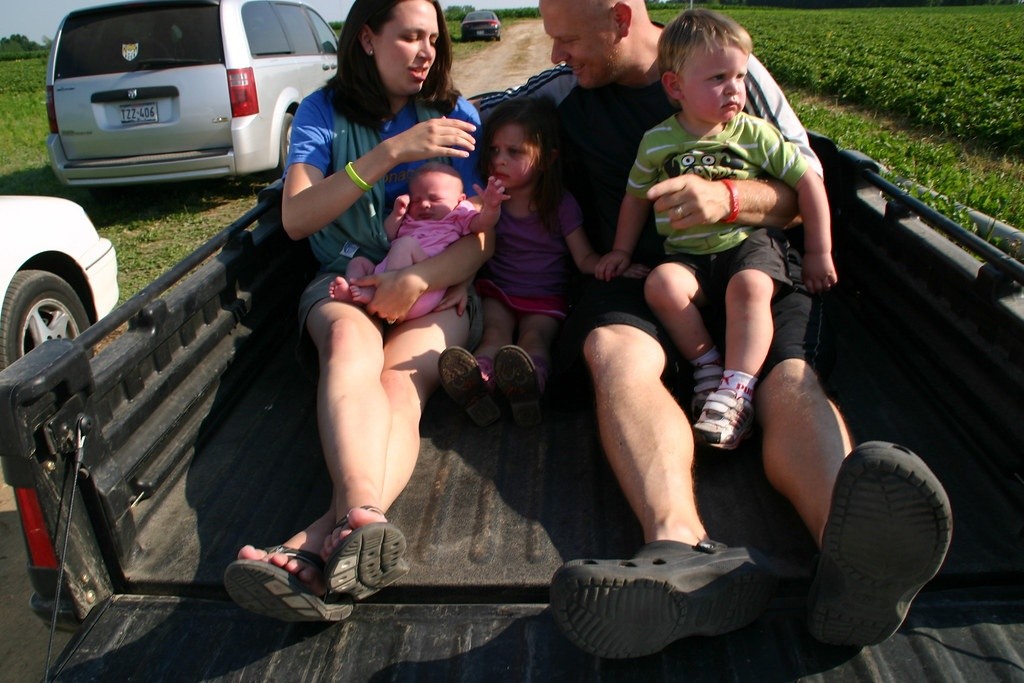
[220,545,357,622]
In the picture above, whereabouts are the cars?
[0,193,123,378]
[460,10,501,41]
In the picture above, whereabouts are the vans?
[42,0,347,198]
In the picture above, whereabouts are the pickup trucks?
[0,82,1024,683]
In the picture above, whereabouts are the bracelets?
[721,177,739,224]
[344,161,374,193]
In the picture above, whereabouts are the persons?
[219,0,497,624]
[439,98,650,430]
[538,0,952,661]
[593,8,838,448]
[328,161,512,323]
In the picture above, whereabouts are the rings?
[673,207,683,219]
[386,319,396,325]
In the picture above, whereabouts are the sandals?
[326,503,410,602]
[806,440,954,651]
[546,539,764,660]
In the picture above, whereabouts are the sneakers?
[692,387,756,455]
[492,343,545,433]
[691,363,725,419]
[435,345,502,429]
